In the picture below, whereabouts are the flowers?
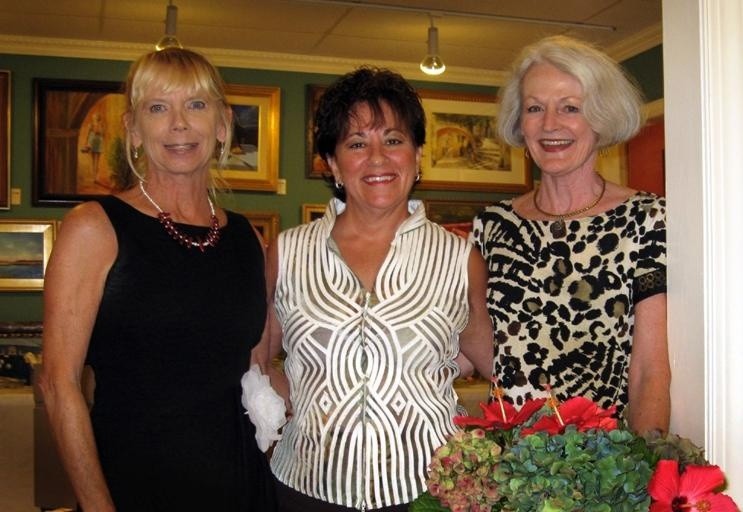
[404,374,736,512]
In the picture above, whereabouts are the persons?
[39,48,290,512]
[84,114,104,182]
[267,68,494,512]
[455,35,672,438]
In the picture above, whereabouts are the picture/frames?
[233,209,281,246]
[2,218,64,292]
[1,70,12,211]
[210,85,280,195]
[421,197,495,242]
[415,89,531,193]
[30,73,130,206]
[304,82,334,181]
[300,202,329,225]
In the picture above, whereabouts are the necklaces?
[139,177,222,252]
[534,171,606,218]
